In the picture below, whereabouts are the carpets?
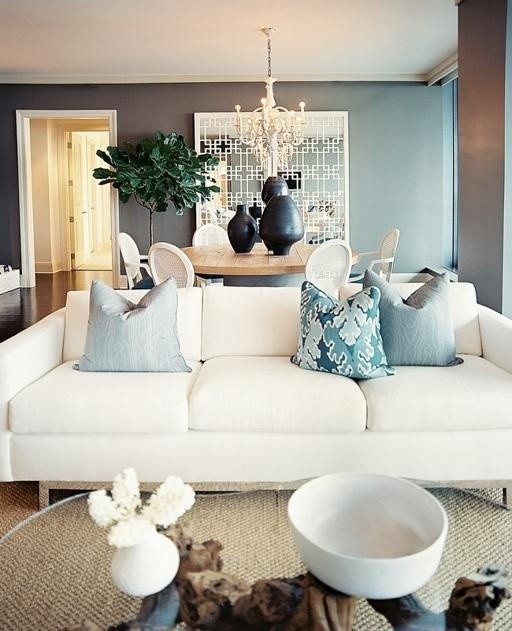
[0,480,512,631]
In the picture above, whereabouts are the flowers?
[81,468,199,549]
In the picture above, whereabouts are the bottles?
[224,175,305,256]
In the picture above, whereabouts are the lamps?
[235,25,310,171]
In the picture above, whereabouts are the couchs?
[0,288,512,514]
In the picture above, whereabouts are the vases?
[105,534,183,598]
[227,175,306,256]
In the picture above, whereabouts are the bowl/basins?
[285,470,451,601]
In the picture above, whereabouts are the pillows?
[289,269,464,380]
[72,277,192,374]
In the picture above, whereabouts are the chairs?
[113,222,401,286]
